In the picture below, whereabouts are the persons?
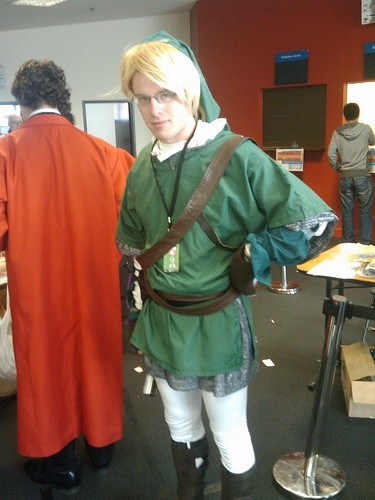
[327,103,375,245]
[7,113,23,132]
[116,31,339,500]
[0,60,137,496]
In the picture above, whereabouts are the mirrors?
[82,100,136,156]
[0,102,21,137]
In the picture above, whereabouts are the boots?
[219,462,256,500]
[170,433,208,500]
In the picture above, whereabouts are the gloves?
[230,245,256,295]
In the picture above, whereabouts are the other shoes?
[24,461,80,489]
[95,455,114,474]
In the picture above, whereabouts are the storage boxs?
[339,341,375,418]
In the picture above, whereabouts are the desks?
[295,242,375,390]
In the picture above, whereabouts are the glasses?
[131,91,176,106]
